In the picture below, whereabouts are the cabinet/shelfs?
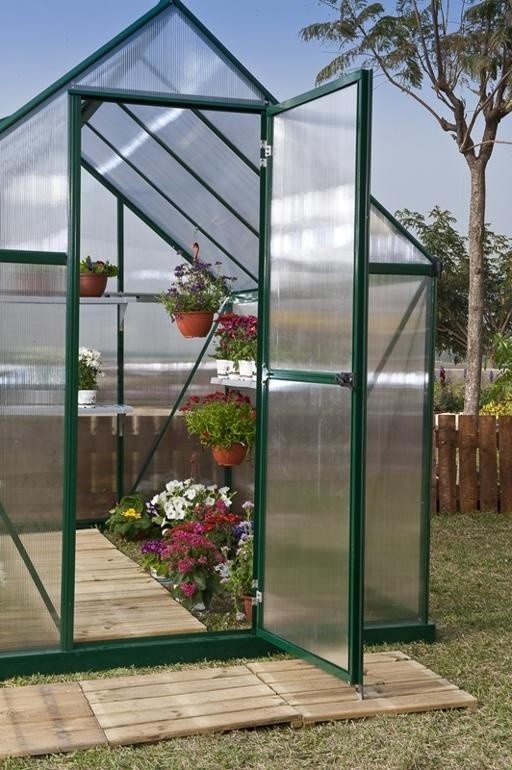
[0,294,137,417]
